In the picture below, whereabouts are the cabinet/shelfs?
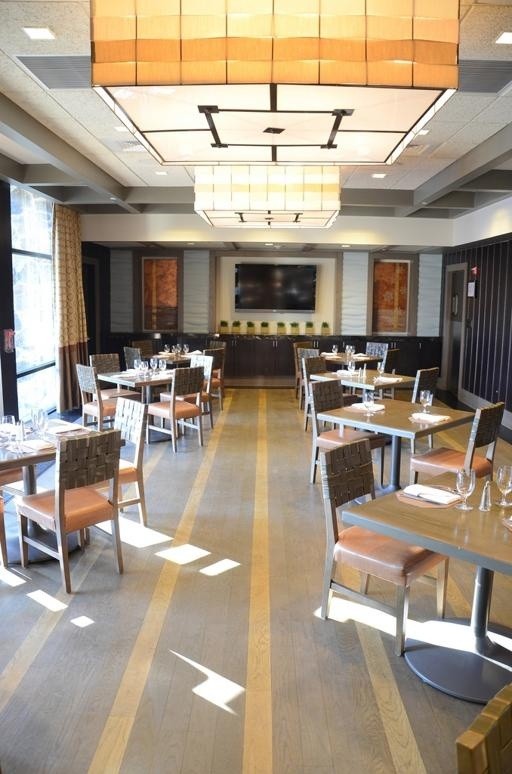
[105,332,439,379]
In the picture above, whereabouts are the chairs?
[75,363,118,432]
[209,340,227,398]
[145,365,205,453]
[410,366,441,455]
[123,347,147,390]
[319,437,450,658]
[292,340,313,399]
[159,354,215,435]
[89,353,142,428]
[296,347,320,410]
[308,378,395,490]
[201,347,225,413]
[301,356,358,433]
[131,339,154,362]
[362,348,400,401]
[451,678,511,774]
[409,401,505,486]
[1,462,38,487]
[363,341,390,371]
[16,428,124,598]
[85,396,148,545]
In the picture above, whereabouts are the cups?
[495,465,512,506]
[377,361,384,377]
[150,358,157,373]
[172,345,177,352]
[2,414,16,445]
[159,359,166,373]
[133,358,141,375]
[332,344,338,355]
[362,391,375,416]
[348,360,355,373]
[454,467,476,510]
[31,406,41,434]
[419,389,433,413]
[141,360,149,377]
[183,344,189,354]
[164,344,170,352]
[36,410,49,439]
[345,344,355,356]
[11,419,26,452]
[177,343,183,352]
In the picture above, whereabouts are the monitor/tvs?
[234,262,317,314]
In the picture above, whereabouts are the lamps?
[88,3,461,167]
[193,166,343,232]
